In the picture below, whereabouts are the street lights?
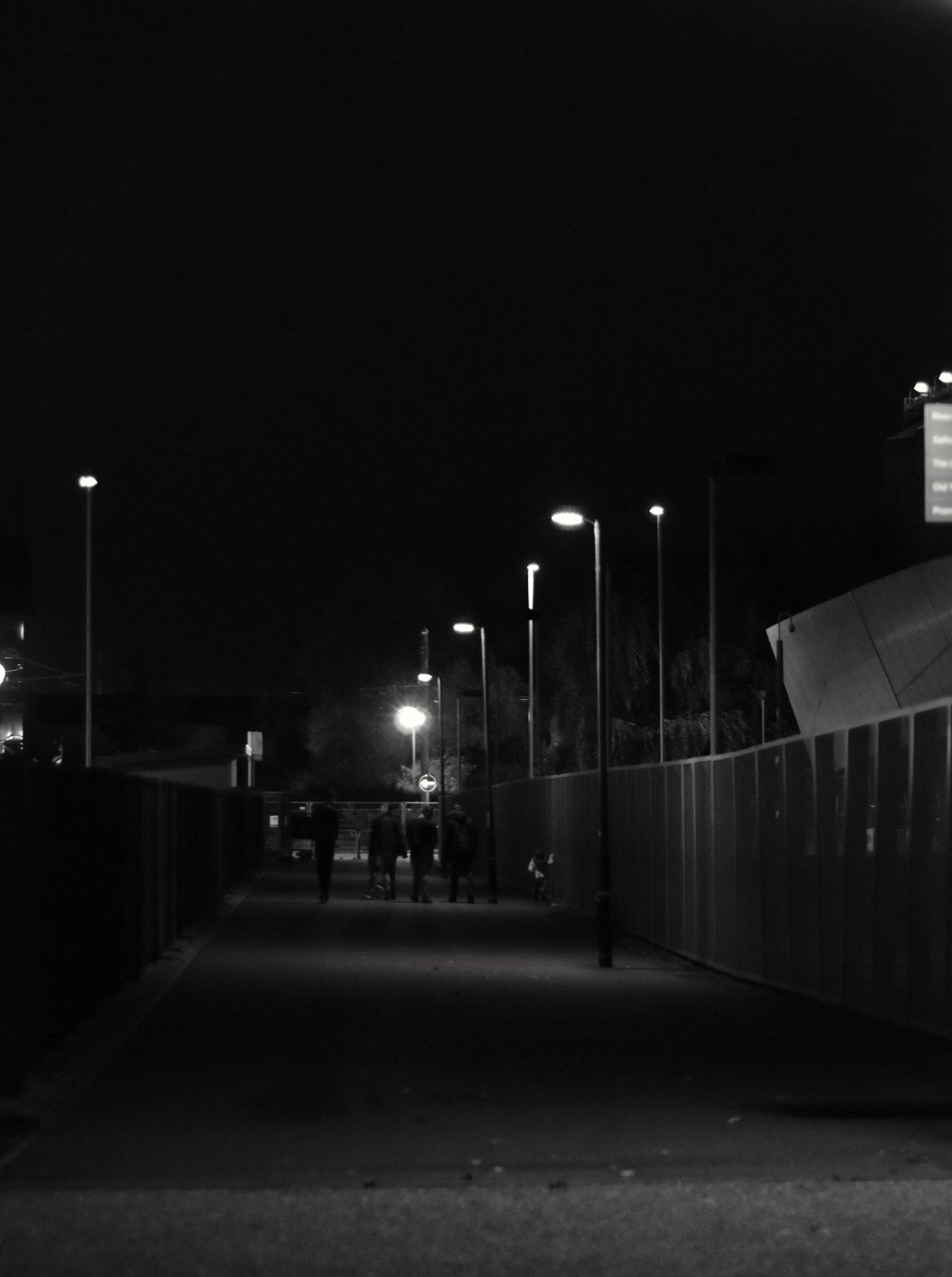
[645,502,667,761]
[524,558,542,778]
[451,620,497,903]
[77,472,97,764]
[550,506,616,970]
[397,705,427,797]
[418,672,448,877]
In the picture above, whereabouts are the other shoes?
[468,898,474,903]
[423,899,432,903]
[449,897,456,902]
[321,898,328,903]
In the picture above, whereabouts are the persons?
[406,809,439,903]
[311,788,338,901]
[367,803,407,899]
[444,812,478,904]
